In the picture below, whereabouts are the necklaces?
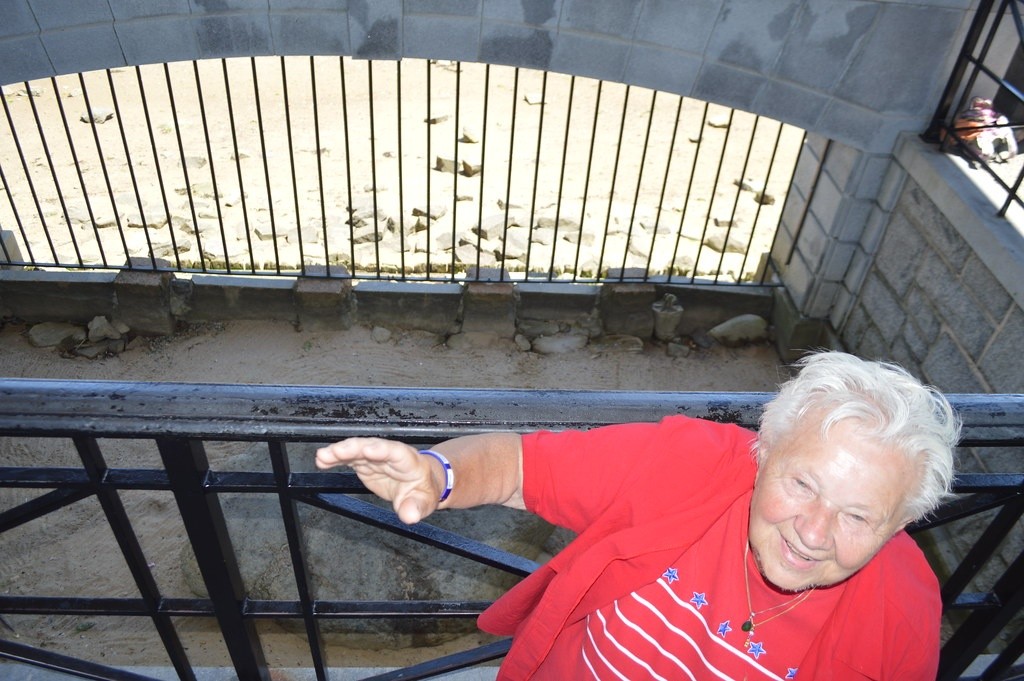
[742,537,815,632]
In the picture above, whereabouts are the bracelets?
[419,447,455,504]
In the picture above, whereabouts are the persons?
[315,349,964,681]
